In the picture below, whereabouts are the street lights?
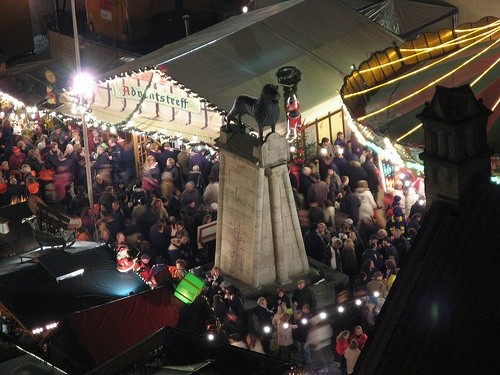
[74,72,94,208]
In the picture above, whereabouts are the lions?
[226,84,280,144]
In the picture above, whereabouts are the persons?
[0,89,426,375]
